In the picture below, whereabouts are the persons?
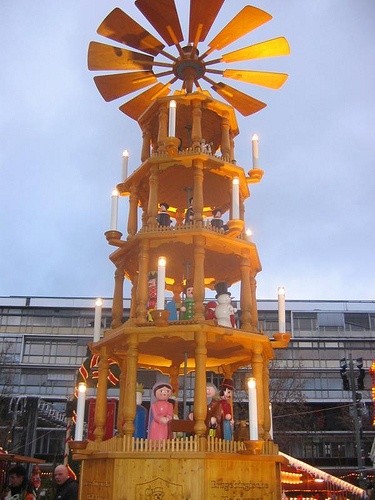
[205,381,223,438]
[210,209,224,229]
[186,197,195,227]
[220,378,236,440]
[8,464,39,500]
[157,202,171,229]
[200,138,212,155]
[179,279,198,320]
[150,381,175,440]
[132,382,148,440]
[148,272,158,322]
[52,464,79,500]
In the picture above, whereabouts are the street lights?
[339,353,368,490]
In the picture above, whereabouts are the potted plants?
[136,382,144,406]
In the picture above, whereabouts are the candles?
[93,298,101,344]
[121,148,129,183]
[75,382,86,442]
[168,100,177,139]
[248,378,259,442]
[252,133,260,171]
[279,286,287,333]
[111,190,119,230]
[231,178,240,219]
[156,257,165,312]
[269,404,273,439]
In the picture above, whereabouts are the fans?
[88,0,291,131]
